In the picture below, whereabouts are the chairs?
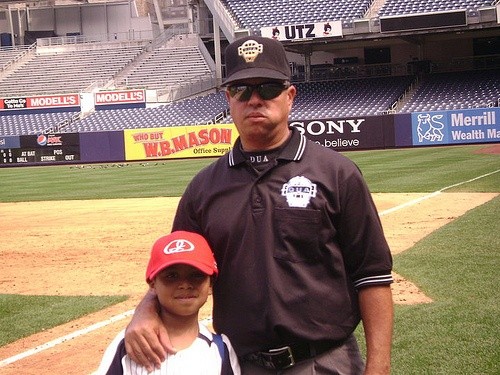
[0,63,500,136]
[222,0,500,36]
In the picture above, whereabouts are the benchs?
[0,45,212,99]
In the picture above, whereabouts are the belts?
[240,338,345,372]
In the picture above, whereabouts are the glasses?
[224,80,289,102]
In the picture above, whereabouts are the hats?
[220,36,291,87]
[146,231,219,282]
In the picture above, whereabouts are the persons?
[124,35,394,375]
[97,230,242,375]
